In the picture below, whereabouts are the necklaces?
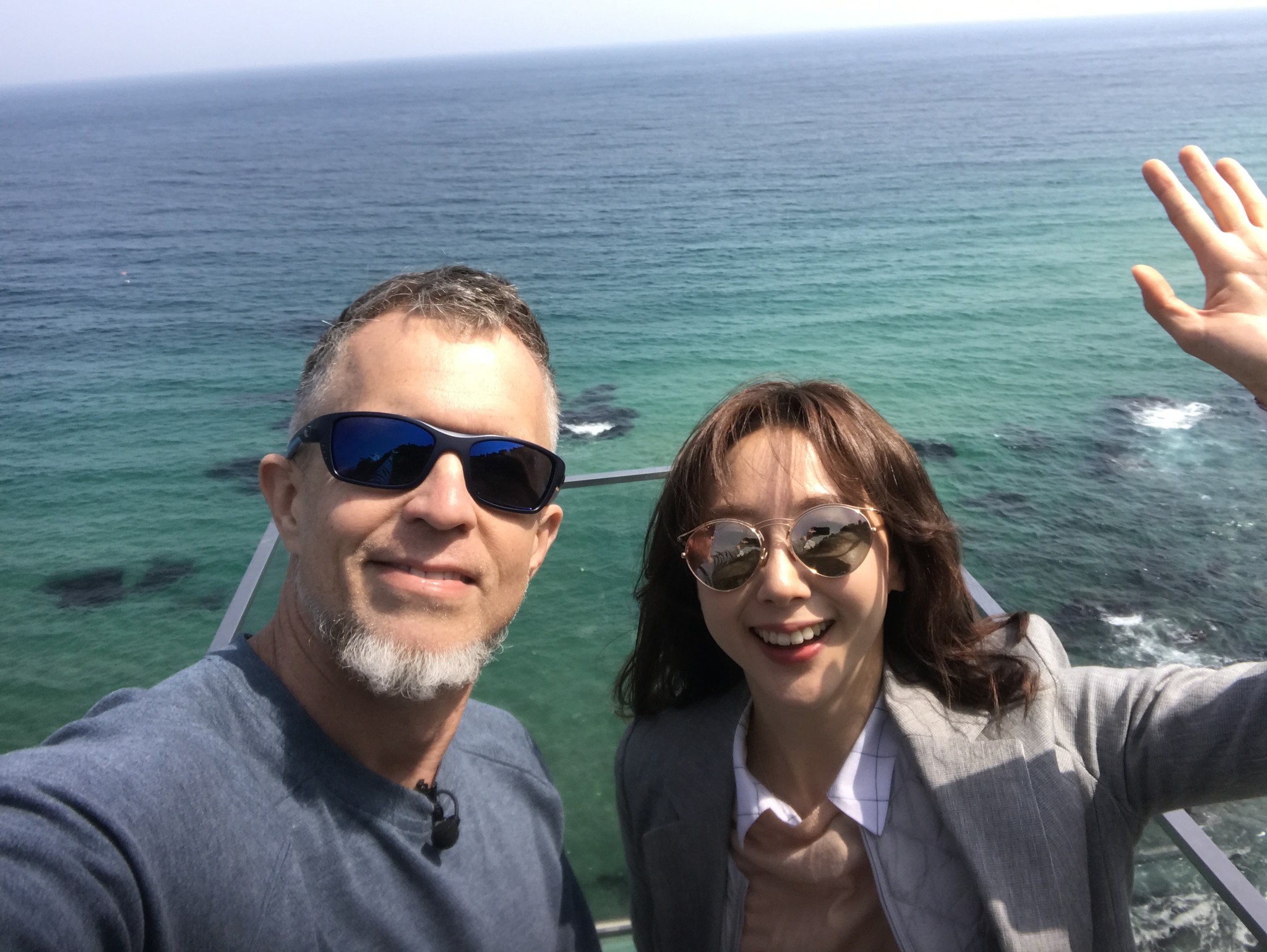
[748,726,771,790]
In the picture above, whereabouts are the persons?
[611,146,1267,952]
[686,524,748,588]
[367,448,400,485]
[791,527,816,556]
[0,266,604,952]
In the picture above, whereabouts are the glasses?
[678,501,888,593]
[286,410,565,513]
